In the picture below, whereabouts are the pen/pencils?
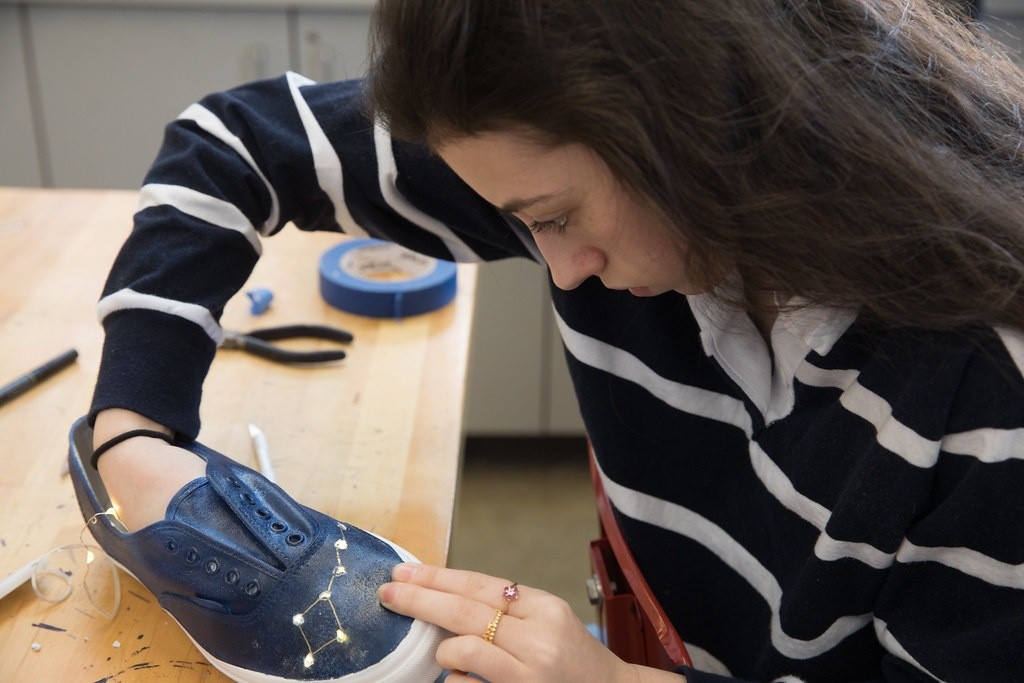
[248,422,277,486]
[0,349,79,404]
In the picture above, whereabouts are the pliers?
[220,323,354,364]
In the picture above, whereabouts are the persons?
[88,0,1024,683]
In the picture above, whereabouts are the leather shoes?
[68,416,443,683]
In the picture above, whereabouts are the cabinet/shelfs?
[0,0,584,435]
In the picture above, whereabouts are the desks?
[0,186,482,683]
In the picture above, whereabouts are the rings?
[500,582,522,615]
[484,607,505,644]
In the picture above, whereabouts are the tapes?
[319,238,459,320]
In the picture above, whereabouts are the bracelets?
[89,429,178,474]
